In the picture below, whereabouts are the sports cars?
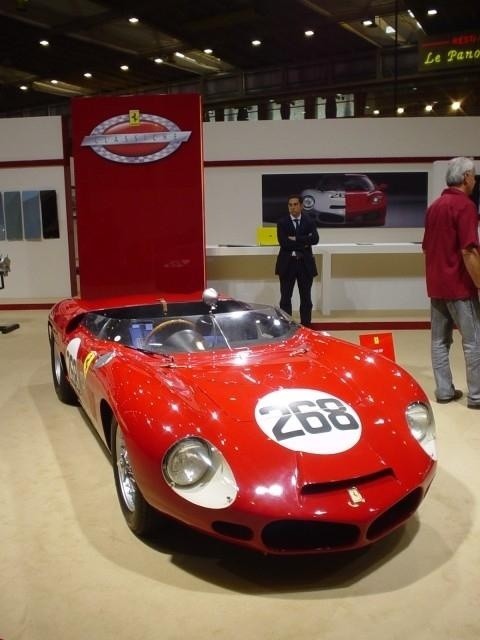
[300,174,390,228]
[46,285,439,557]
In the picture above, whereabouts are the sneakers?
[437,384,462,403]
[468,404,480,409]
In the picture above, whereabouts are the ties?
[294,219,299,230]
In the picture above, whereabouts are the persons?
[274,194,319,330]
[421,157,480,411]
